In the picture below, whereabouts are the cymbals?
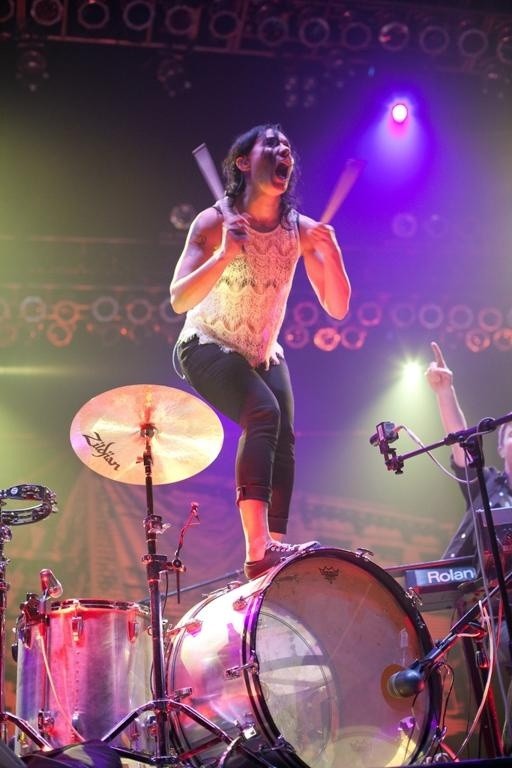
[70,386,223,486]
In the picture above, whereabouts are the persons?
[169,124,351,581]
[426,341,512,668]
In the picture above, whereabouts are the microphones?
[387,670,425,698]
[190,502,199,517]
[369,420,402,447]
[39,569,64,600]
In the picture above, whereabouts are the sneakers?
[245,537,320,581]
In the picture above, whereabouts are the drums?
[167,547,441,765]
[15,600,167,767]
